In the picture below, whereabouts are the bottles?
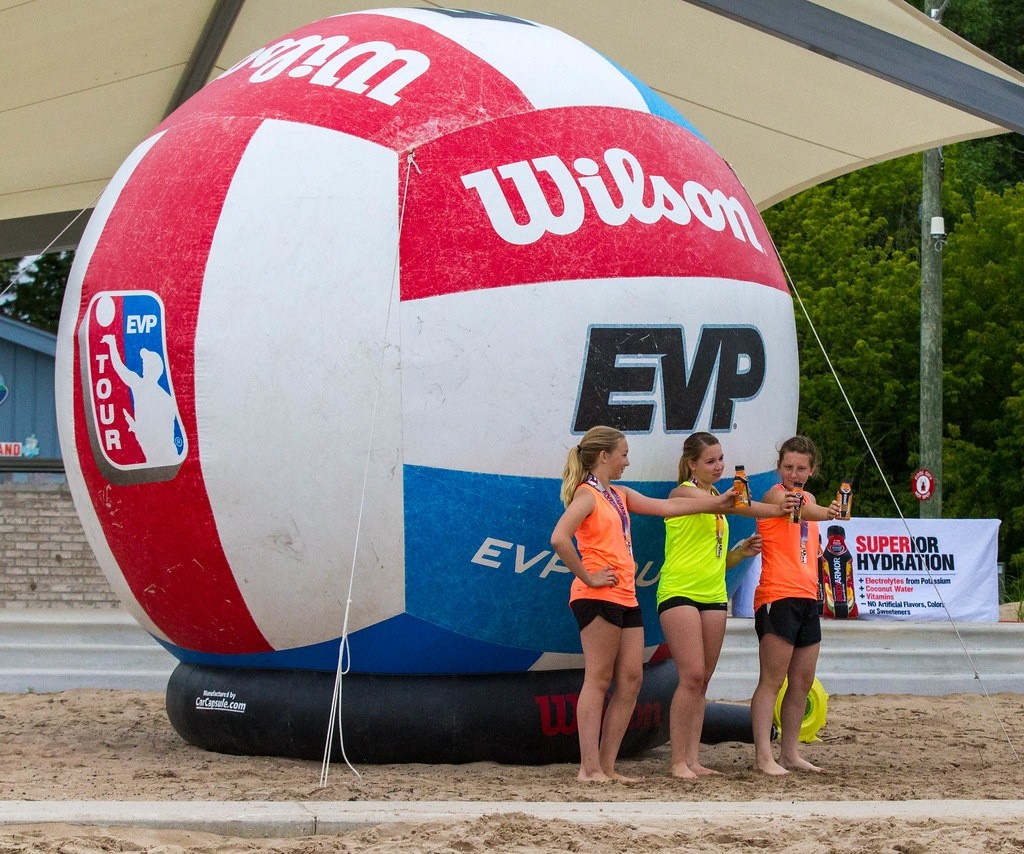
[835,478,853,520]
[817,525,858,619]
[786,481,804,524]
[733,465,751,509]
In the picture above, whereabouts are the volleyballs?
[53,5,804,677]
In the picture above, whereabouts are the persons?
[750,436,841,775]
[550,425,752,782]
[656,432,805,779]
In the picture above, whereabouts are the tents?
[0,0,1024,260]
